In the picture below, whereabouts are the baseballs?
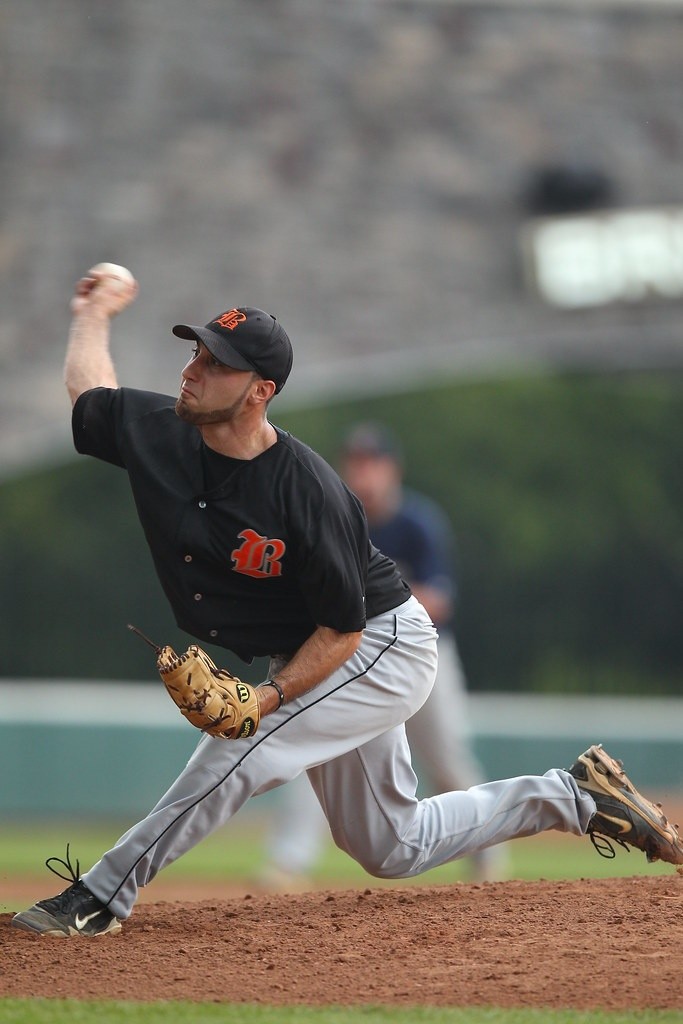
[91,261,133,284]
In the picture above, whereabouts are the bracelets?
[262,680,284,712]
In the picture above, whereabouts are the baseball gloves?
[157,643,261,740]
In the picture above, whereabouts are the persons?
[254,423,506,882]
[12,263,683,935]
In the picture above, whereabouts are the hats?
[172,309,293,395]
[344,423,397,459]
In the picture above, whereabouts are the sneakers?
[564,743,683,873]
[11,843,122,940]
